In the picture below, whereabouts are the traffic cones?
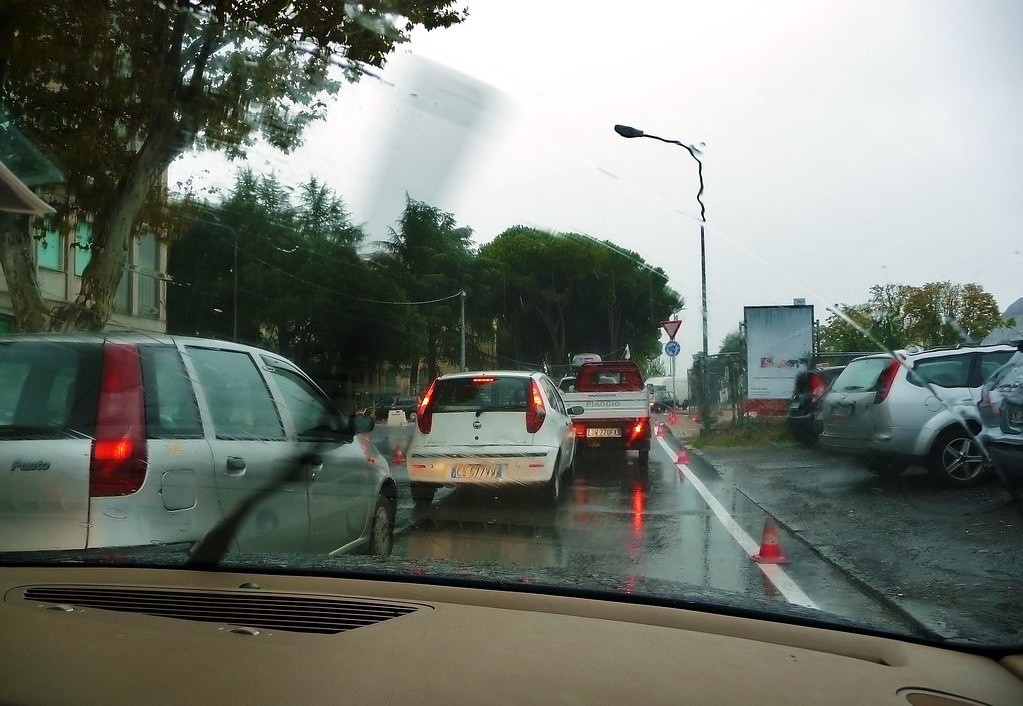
[654,423,667,437]
[673,443,691,465]
[750,516,793,566]
[390,443,405,464]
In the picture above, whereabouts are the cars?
[557,374,616,393]
[362,395,422,422]
[405,366,585,508]
[649,390,680,413]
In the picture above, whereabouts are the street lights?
[614,122,710,430]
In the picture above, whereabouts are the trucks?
[558,358,656,462]
[643,374,692,411]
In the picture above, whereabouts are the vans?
[0,330,400,565]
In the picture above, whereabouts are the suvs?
[815,341,1019,490]
[784,362,849,435]
[975,338,1023,501]
